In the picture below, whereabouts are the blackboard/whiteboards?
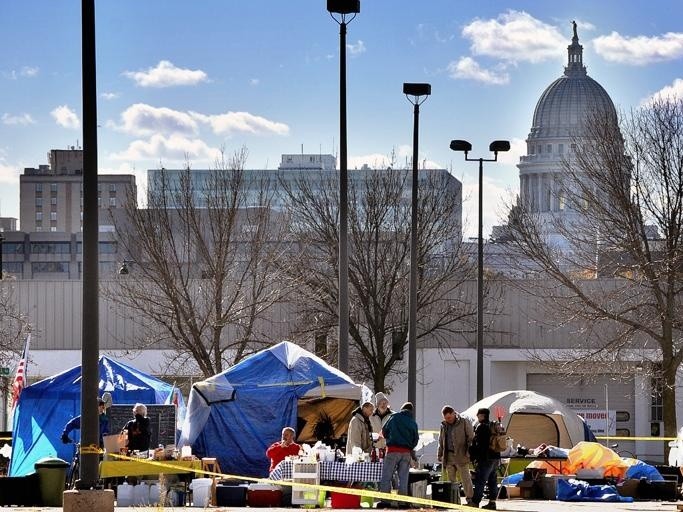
[110,404,176,449]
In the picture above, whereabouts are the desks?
[99,460,203,508]
[498,456,568,475]
[268,459,401,508]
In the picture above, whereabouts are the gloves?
[61,435,68,444]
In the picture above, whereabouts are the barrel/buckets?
[135,482,148,504]
[431,481,460,503]
[117,481,133,507]
[534,476,555,499]
[331,491,360,508]
[149,483,162,504]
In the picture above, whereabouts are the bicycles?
[60,438,80,489]
[610,444,635,459]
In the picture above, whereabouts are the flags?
[9,330,31,407]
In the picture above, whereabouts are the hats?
[375,393,388,406]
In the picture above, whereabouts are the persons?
[120,403,150,452]
[59,396,110,446]
[461,408,500,510]
[264,426,299,474]
[376,401,418,508]
[437,405,475,502]
[346,401,373,488]
[368,390,396,434]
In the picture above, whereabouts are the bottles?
[379,448,384,458]
[370,446,376,461]
[517,443,521,453]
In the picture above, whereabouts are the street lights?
[450,140,510,401]
[327,0,359,375]
[404,82,431,422]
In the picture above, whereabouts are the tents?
[185,340,372,484]
[5,353,184,477]
[457,388,601,452]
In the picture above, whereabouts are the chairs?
[493,458,512,500]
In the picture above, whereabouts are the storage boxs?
[575,466,606,479]
[216,483,283,507]
[541,473,576,499]
[515,481,542,499]
[330,484,363,508]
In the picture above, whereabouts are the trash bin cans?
[34,457,71,507]
[409,469,430,508]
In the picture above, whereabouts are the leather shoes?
[482,501,496,510]
[463,501,479,508]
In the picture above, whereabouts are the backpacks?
[486,421,508,451]
